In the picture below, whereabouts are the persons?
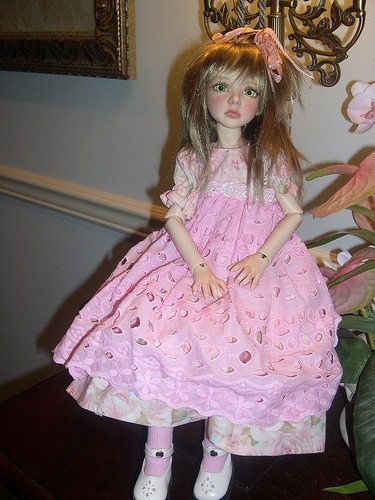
[53,27,344,500]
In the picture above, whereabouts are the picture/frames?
[0,0,138,79]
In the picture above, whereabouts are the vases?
[338,333,368,453]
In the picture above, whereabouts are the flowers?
[305,79,375,500]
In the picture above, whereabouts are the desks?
[0,361,375,500]
